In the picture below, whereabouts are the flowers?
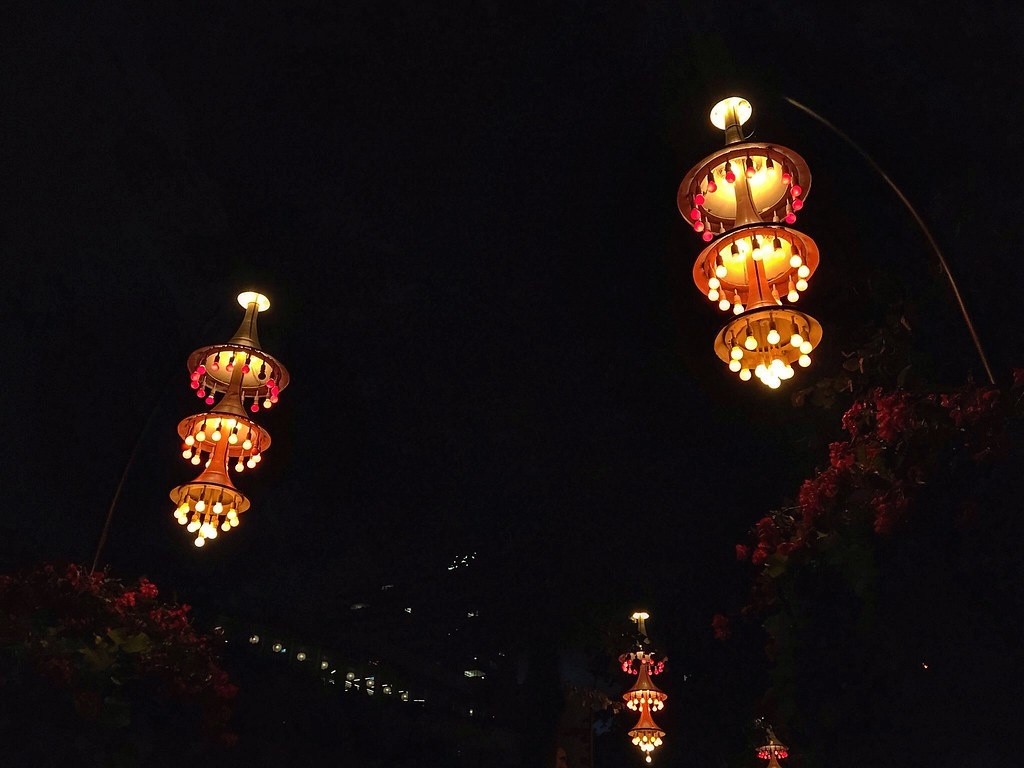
[710,344,1024,768]
[0,560,240,768]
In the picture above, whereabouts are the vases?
[798,521,1024,768]
[0,683,193,768]
